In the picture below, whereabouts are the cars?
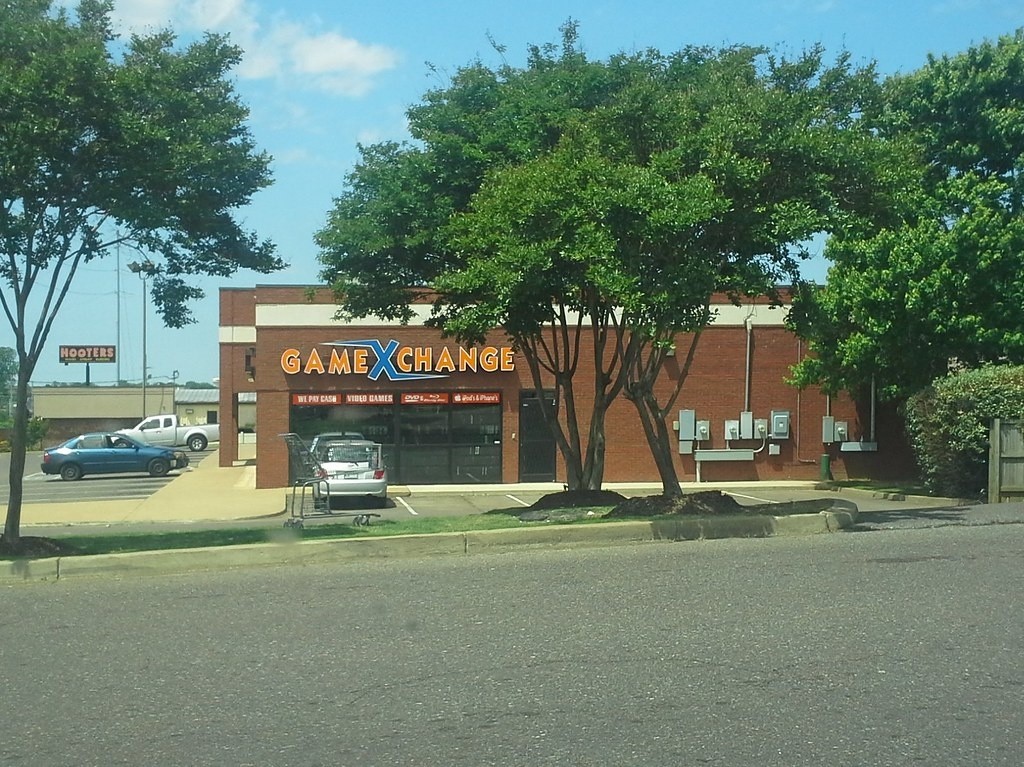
[308,432,369,460]
[40,432,192,482]
[309,441,388,509]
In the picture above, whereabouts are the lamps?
[247,375,254,383]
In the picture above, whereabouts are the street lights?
[127,261,155,416]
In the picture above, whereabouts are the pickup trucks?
[108,414,221,453]
